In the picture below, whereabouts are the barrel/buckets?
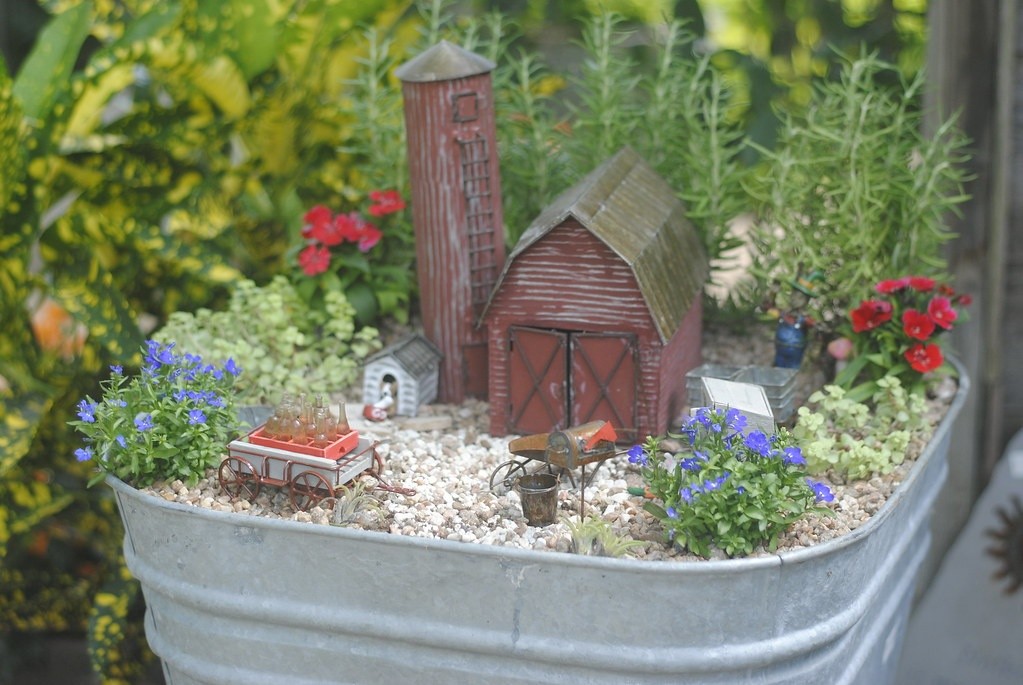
[547,419,616,455]
[514,472,560,528]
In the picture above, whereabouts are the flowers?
[65,3,990,560]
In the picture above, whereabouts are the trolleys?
[491,418,673,524]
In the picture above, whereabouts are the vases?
[96,346,978,685]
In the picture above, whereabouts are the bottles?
[265,392,350,449]
